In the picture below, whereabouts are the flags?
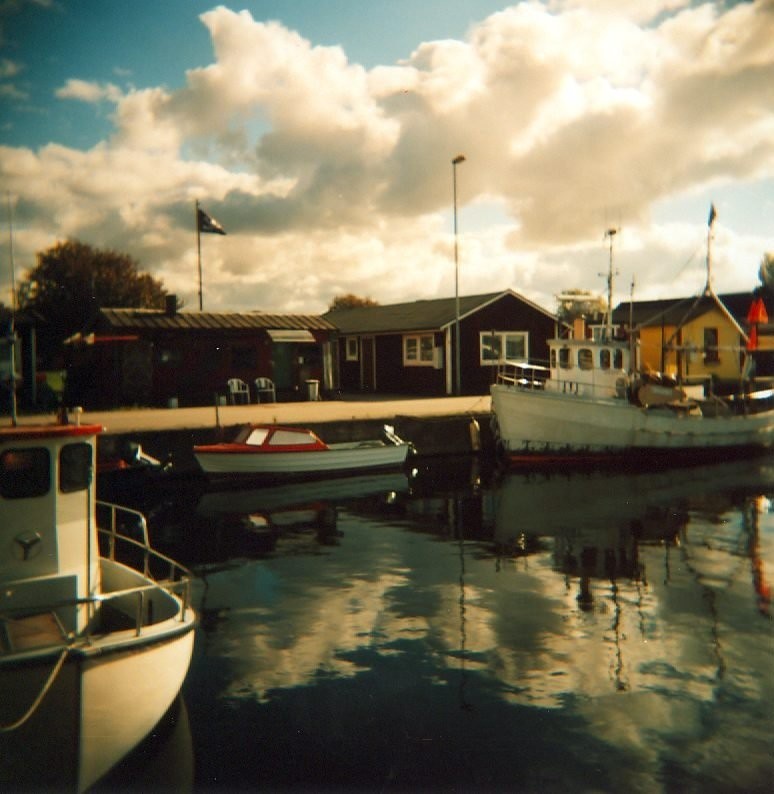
[197,208,228,235]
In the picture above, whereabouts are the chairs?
[254,377,275,403]
[227,378,250,404]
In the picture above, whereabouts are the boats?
[0,330,203,793]
[197,468,413,551]
[477,228,773,459]
[189,418,420,483]
[483,446,773,693]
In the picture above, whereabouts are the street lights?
[452,153,474,397]
